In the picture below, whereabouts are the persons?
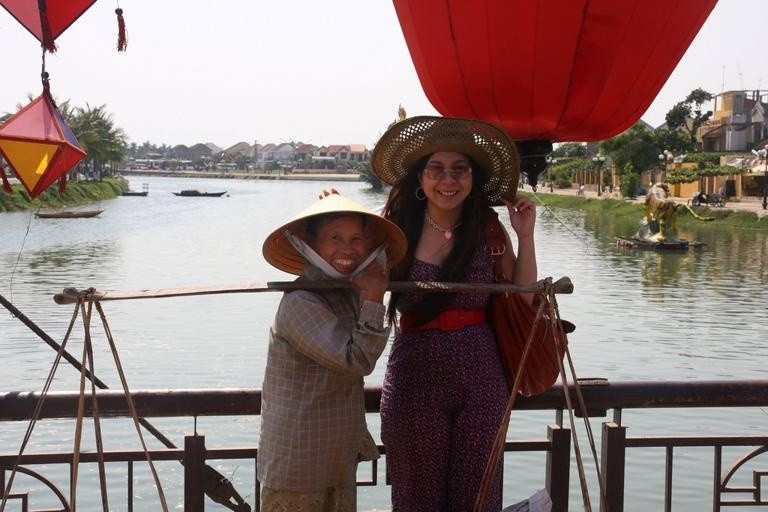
[256,196,412,512]
[315,114,540,512]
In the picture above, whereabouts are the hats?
[261,191,410,279]
[366,114,524,207]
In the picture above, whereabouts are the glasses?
[420,165,474,182]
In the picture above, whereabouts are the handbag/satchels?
[488,280,576,399]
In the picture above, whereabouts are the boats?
[171,190,228,196]
[122,191,149,196]
[33,209,105,219]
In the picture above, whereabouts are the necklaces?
[422,208,464,239]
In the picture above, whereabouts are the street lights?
[592,152,606,196]
[758,144,767,210]
[546,156,558,193]
[658,149,674,183]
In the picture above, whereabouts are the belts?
[398,308,487,333]
